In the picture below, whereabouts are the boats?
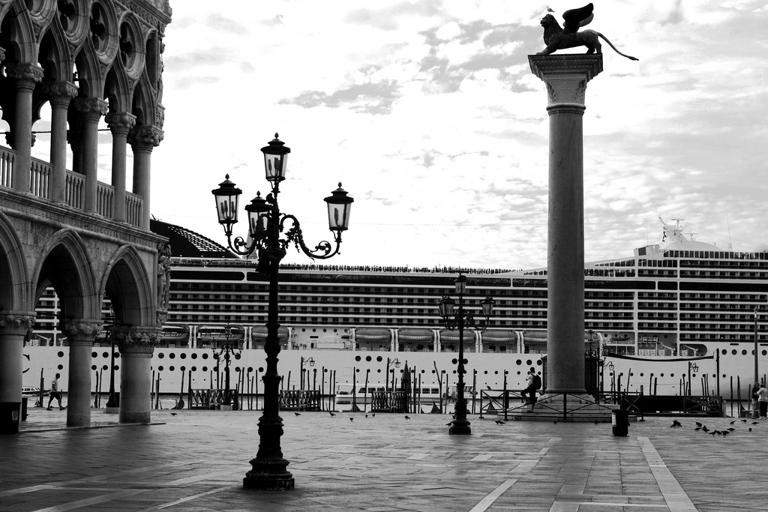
[334,381,473,405]
[21,385,52,397]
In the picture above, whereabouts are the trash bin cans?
[22,398,27,420]
[611,409,628,436]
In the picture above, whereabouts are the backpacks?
[532,373,541,389]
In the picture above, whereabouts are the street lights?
[385,357,401,407]
[438,274,497,435]
[688,360,698,396]
[600,361,614,397]
[210,133,355,491]
[301,356,315,403]
[753,306,760,384]
[101,305,121,393]
[210,321,243,410]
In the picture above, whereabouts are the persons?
[750,382,758,418]
[520,368,538,413]
[47,373,67,411]
[756,384,768,419]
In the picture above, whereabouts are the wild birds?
[171,412,177,416]
[372,414,375,416]
[694,420,736,438]
[749,427,752,432]
[494,419,506,425]
[365,414,368,418]
[329,411,336,417]
[405,416,410,419]
[751,421,759,425]
[741,419,747,423]
[350,417,354,422]
[295,412,301,416]
[670,420,683,428]
[545,6,555,14]
[446,422,451,426]
